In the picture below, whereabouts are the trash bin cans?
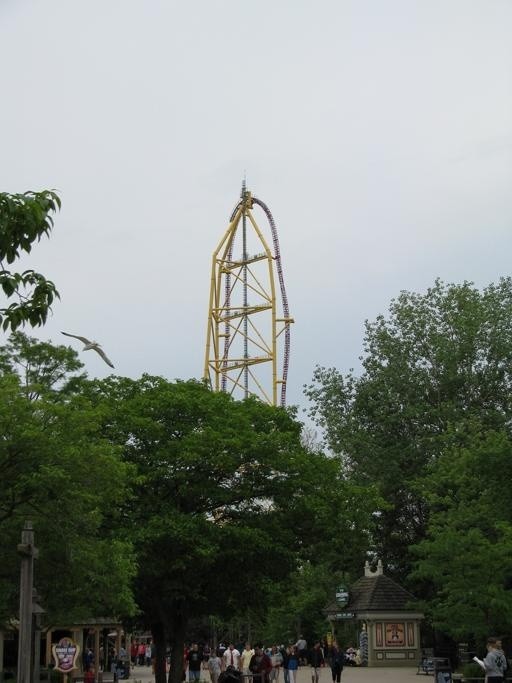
[433,658,452,683]
[112,655,130,679]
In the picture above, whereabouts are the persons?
[495,639,510,682]
[83,634,361,683]
[483,636,507,682]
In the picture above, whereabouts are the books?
[472,656,486,670]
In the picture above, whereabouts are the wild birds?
[61,332,116,370]
